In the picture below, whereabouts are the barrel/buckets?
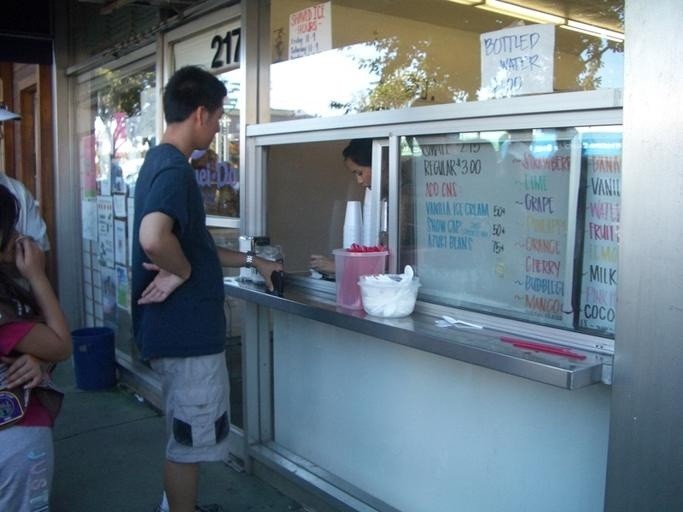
[333,247,387,312]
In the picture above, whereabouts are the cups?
[363,186,380,248]
[341,201,362,251]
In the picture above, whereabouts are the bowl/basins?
[356,273,422,320]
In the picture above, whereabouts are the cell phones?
[265,269,285,297]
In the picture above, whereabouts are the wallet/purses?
[265,271,288,297]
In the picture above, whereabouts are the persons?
[308,140,415,282]
[129,66,284,511]
[0,184,74,510]
[404,94,587,166]
[189,148,240,219]
[0,108,53,324]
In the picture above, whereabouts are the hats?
[0,108,24,121]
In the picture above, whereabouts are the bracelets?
[245,251,256,269]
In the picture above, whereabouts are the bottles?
[252,236,271,284]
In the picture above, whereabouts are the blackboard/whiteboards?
[409,133,622,340]
